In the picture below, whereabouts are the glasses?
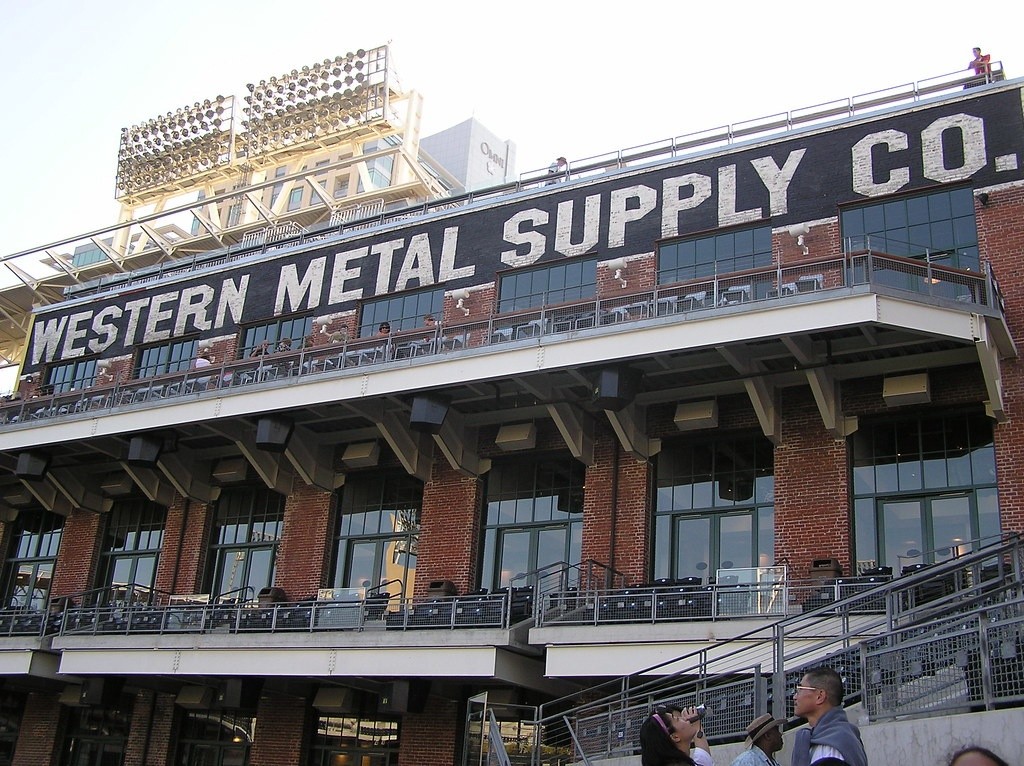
[381,326,390,329]
[655,704,667,714]
[797,684,816,690]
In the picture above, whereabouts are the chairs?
[0,274,823,427]
[582,578,1024,755]
[1,558,1012,637]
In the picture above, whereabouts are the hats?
[743,713,787,750]
[557,157,567,164]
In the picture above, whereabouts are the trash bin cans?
[46,595,74,614]
[808,557,842,587]
[256,586,287,608]
[427,578,457,600]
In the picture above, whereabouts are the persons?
[423,312,441,326]
[792,666,869,766]
[949,745,1010,766]
[650,705,716,766]
[297,335,314,349]
[545,156,568,186]
[968,47,991,75]
[195,351,229,388]
[727,713,788,766]
[273,337,292,355]
[640,712,698,766]
[374,321,391,335]
[249,340,269,358]
[328,323,351,344]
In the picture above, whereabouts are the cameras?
[692,704,708,719]
